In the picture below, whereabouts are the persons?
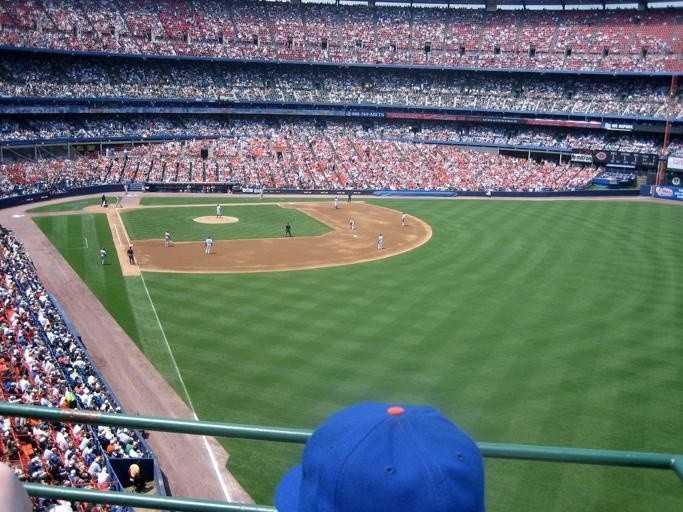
[378,233,383,250]
[400,212,406,225]
[349,219,354,230]
[286,222,291,237]
[216,204,221,218]
[165,231,171,247]
[204,236,212,254]
